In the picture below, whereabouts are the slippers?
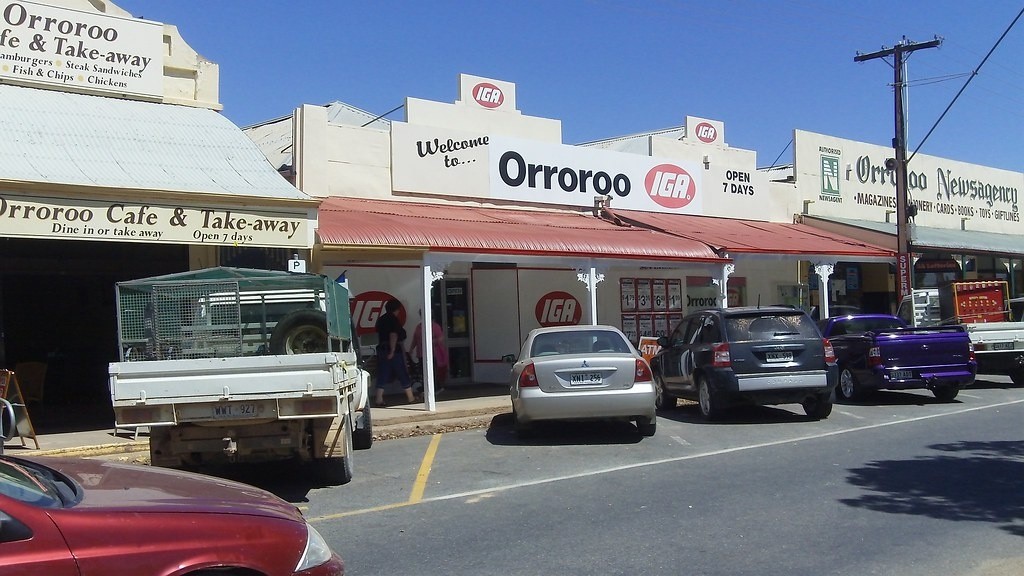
[409,399,421,404]
[374,402,388,407]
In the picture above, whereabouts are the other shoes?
[435,387,444,395]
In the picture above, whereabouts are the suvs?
[649,307,839,423]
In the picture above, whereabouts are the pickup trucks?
[816,315,977,402]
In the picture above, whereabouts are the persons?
[410,308,449,395]
[375,300,420,407]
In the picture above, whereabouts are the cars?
[0,450,347,576]
[502,325,660,436]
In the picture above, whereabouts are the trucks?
[896,286,1024,389]
[109,265,372,484]
